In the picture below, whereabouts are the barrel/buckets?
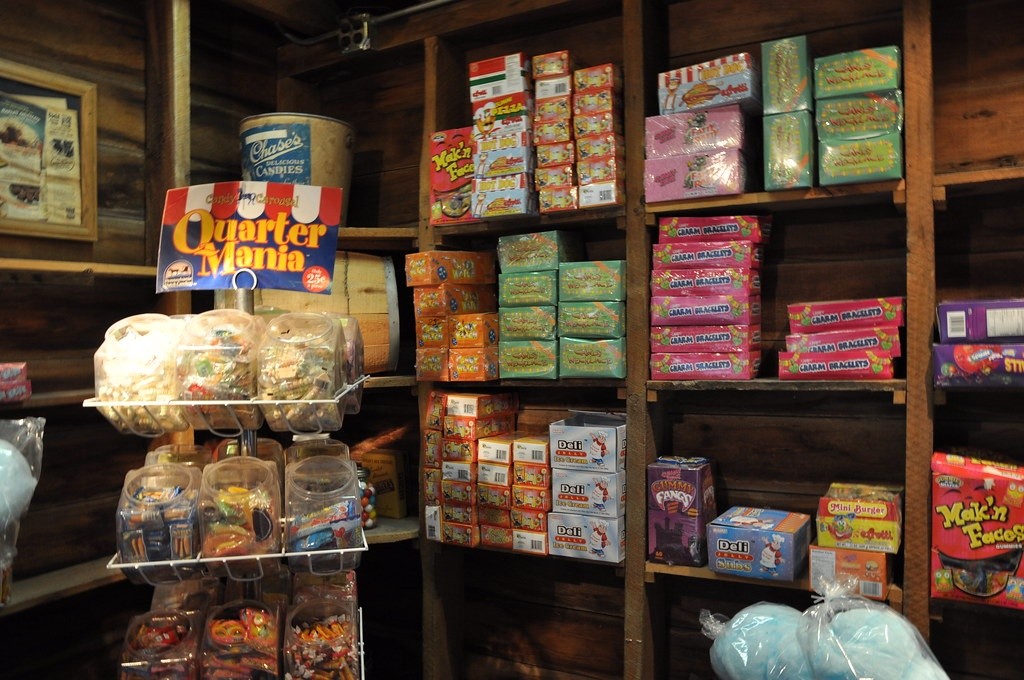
[252,251,399,374]
[238,113,358,226]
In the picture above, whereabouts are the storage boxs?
[347,35,1024,616]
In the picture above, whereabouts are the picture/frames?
[0,57,98,245]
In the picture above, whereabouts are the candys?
[96,320,375,680]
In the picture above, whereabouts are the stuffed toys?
[709,596,949,680]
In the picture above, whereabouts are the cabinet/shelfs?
[0,0,336,680]
[273,0,1024,680]
